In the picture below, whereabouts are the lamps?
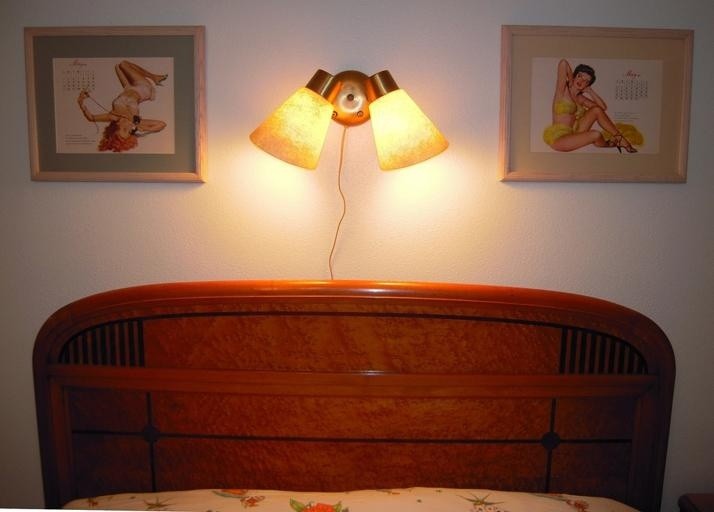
[247,64,452,174]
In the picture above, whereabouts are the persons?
[542,58,640,155]
[76,60,168,153]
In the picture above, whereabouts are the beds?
[29,280,680,510]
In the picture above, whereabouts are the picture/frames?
[23,24,208,185]
[499,23,695,184]
